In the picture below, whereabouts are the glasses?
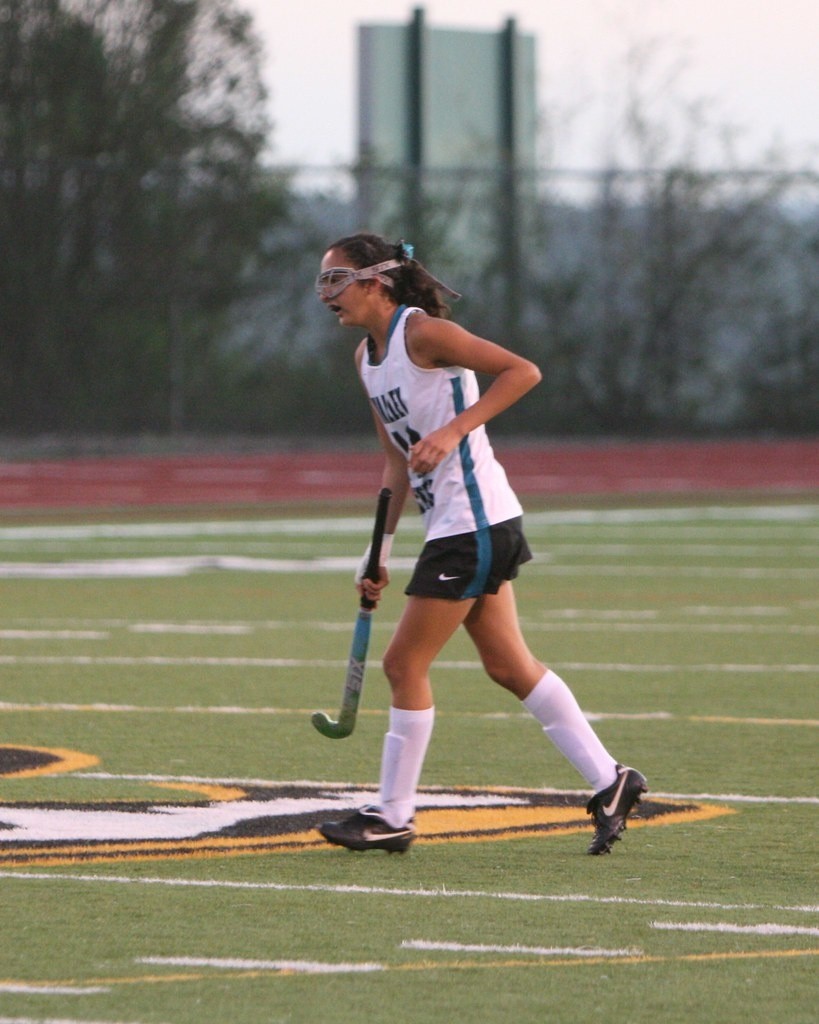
[314,260,404,298]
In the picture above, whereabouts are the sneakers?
[587,765,647,854]
[319,805,419,853]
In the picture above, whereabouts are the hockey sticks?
[313,488,400,739]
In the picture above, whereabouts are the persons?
[318,234,648,857]
